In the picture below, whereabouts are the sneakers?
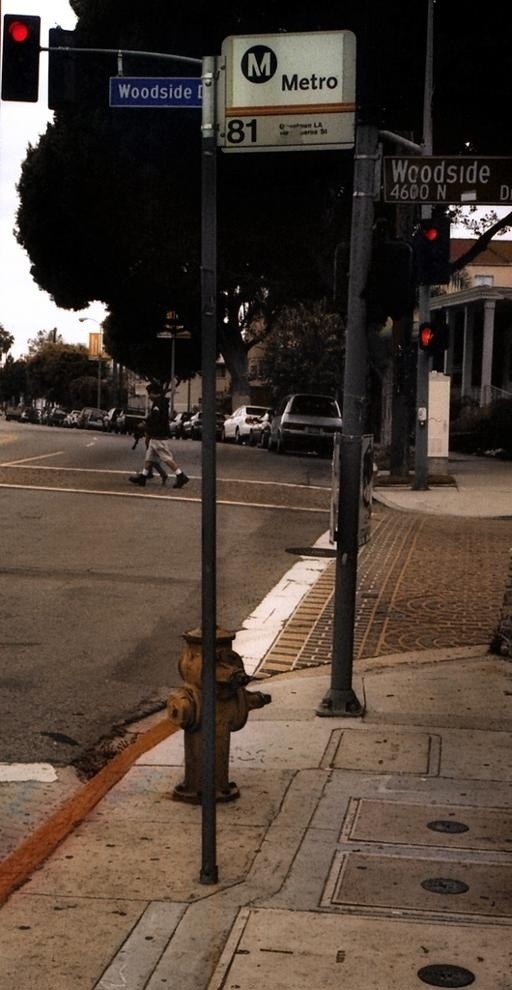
[129,475,147,487]
[139,469,168,484]
[174,473,190,489]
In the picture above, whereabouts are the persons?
[142,421,168,481]
[130,383,189,488]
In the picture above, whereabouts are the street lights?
[78,316,104,415]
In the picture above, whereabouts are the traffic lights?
[414,320,446,354]
[0,10,41,107]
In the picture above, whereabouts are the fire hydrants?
[162,615,270,820]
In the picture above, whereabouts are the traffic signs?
[380,152,512,207]
[110,74,207,111]
[419,218,450,283]
